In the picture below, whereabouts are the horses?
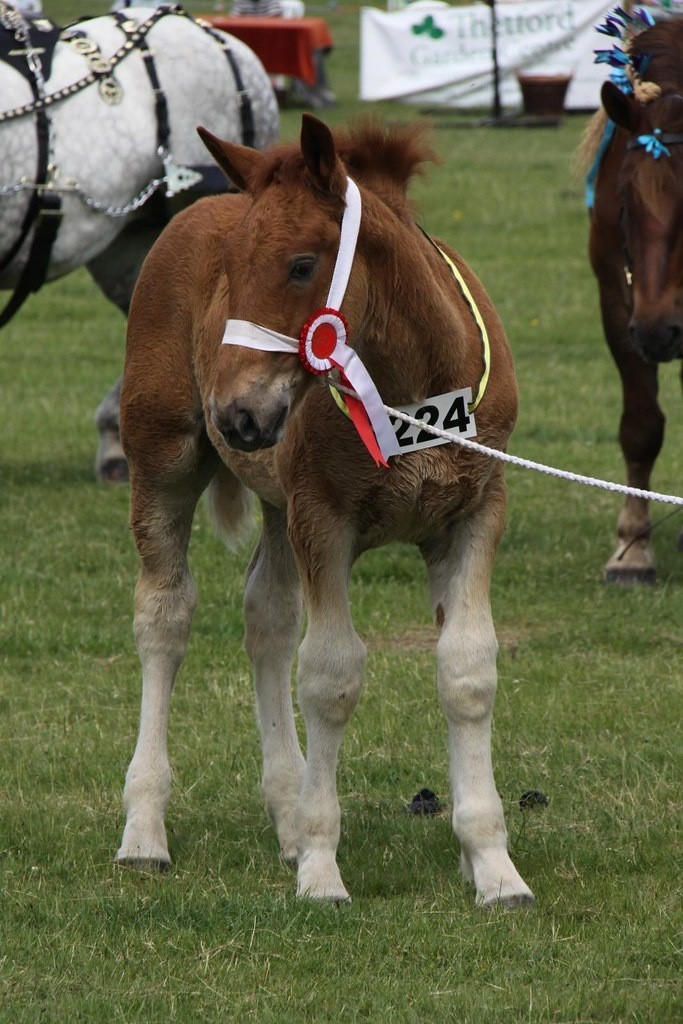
[570,16,683,584]
[0,0,279,483]
[116,115,532,908]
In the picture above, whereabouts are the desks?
[358,0,683,110]
[194,14,337,110]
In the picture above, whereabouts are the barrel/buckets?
[515,67,573,114]
